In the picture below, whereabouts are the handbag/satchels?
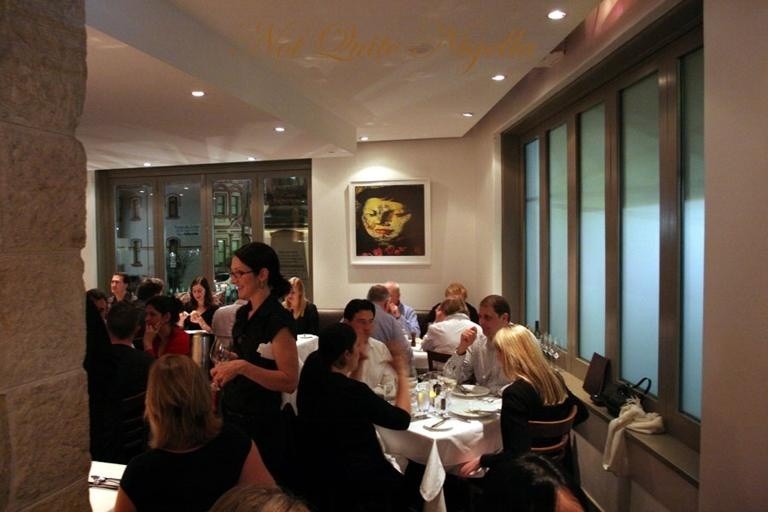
[605,377,651,418]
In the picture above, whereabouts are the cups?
[207,338,234,392]
[417,384,430,412]
[378,384,391,405]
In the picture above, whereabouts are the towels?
[256,341,303,371]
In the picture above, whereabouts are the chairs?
[426,349,452,371]
[527,404,579,478]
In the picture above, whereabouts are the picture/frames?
[348,176,432,266]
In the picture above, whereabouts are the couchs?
[317,307,431,338]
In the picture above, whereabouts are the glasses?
[229,270,254,278]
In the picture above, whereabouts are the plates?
[450,399,498,418]
[451,384,491,397]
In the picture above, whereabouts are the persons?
[83,291,112,381]
[443,295,515,386]
[423,284,479,335]
[343,299,398,388]
[85,288,108,320]
[88,300,150,394]
[105,272,137,320]
[296,323,411,477]
[280,277,319,335]
[383,280,420,340]
[131,279,164,309]
[211,299,248,349]
[176,276,215,333]
[339,284,407,343]
[110,353,223,512]
[460,324,590,483]
[210,241,299,456]
[355,184,424,256]
[421,295,483,371]
[144,296,190,359]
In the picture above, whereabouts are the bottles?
[209,382,224,417]
